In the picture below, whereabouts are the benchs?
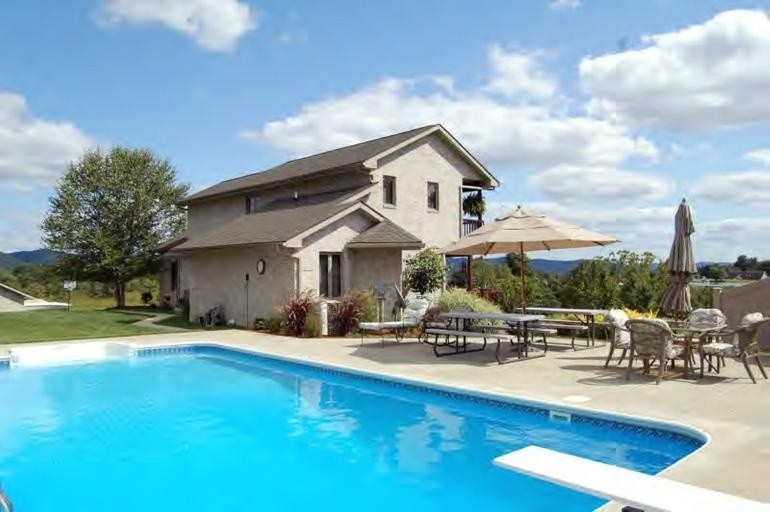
[424,303,615,364]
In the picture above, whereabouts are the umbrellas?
[433,203,621,358]
[659,198,697,332]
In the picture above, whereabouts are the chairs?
[600,304,769,387]
[360,297,432,349]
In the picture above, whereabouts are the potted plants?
[249,289,323,339]
